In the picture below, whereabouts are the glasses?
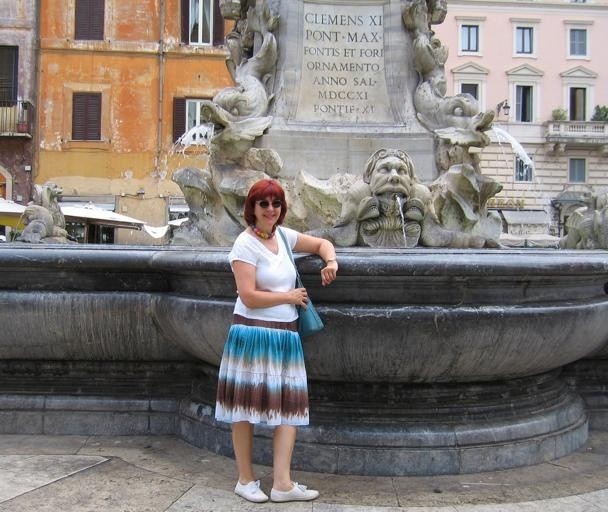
[254,199,282,209]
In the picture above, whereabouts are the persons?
[213,180,340,502]
[363,147,416,219]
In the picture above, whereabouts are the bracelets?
[325,258,337,264]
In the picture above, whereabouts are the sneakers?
[233,478,269,503]
[270,481,321,503]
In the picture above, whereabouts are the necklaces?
[249,222,276,240]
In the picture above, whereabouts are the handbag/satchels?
[293,274,326,338]
